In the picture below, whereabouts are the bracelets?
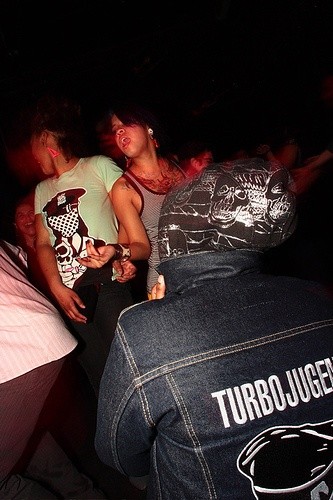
[118,244,131,262]
[107,244,122,260]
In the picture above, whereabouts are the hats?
[159,158,298,262]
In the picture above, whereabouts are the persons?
[0,77,320,500]
[94,156,333,500]
